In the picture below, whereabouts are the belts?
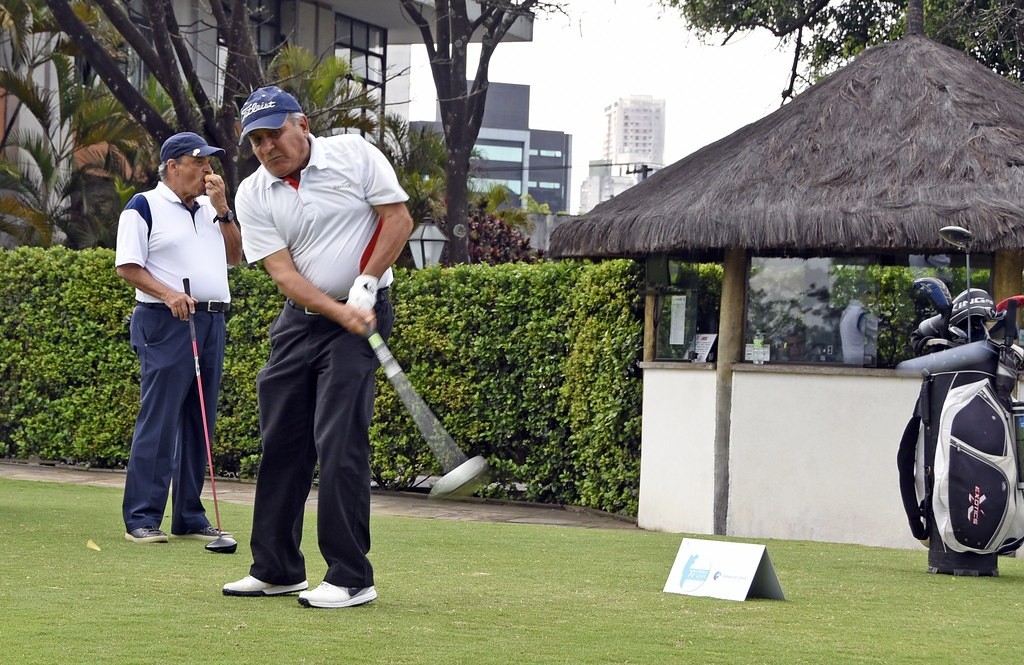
[286,288,389,317]
[136,300,230,313]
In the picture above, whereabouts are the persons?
[112,134,243,543]
[221,86,414,609]
[839,280,879,366]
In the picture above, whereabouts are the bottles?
[753,329,764,365]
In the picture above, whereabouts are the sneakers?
[171,526,233,541]
[297,581,377,608]
[223,574,308,597]
[125,527,168,543]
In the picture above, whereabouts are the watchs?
[212,210,234,223]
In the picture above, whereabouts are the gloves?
[348,275,379,311]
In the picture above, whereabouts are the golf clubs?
[913,226,975,343]
[361,323,490,501]
[183,278,238,554]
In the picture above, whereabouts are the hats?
[160,132,226,165]
[239,85,303,145]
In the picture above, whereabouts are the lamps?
[406,212,449,270]
